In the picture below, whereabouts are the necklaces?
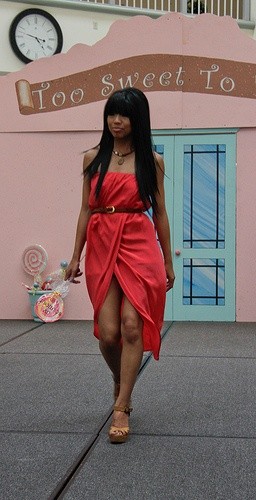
[111,148,135,165]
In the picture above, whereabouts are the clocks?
[10,7,64,64]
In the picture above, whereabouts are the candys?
[34,292,65,323]
[21,244,67,291]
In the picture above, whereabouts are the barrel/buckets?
[28,289,54,323]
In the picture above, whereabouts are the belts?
[91,206,147,214]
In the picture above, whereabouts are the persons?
[64,87,176,443]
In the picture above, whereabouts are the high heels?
[109,405,134,443]
[112,376,120,402]
